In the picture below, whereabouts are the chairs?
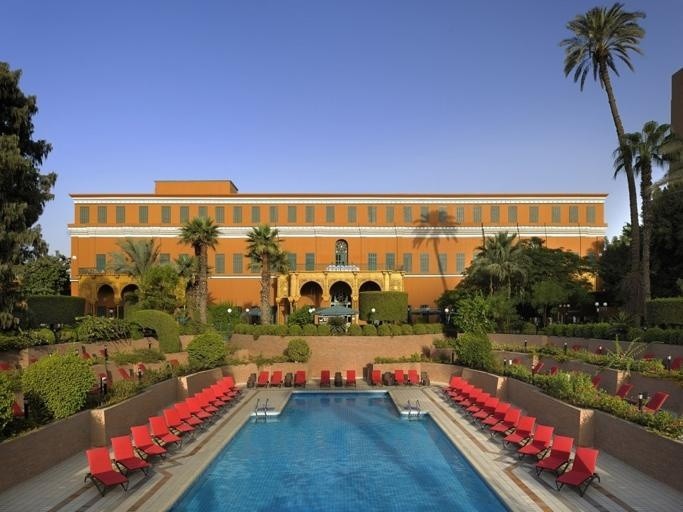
[83,375,242,497]
[246,370,429,388]
[441,376,600,497]
